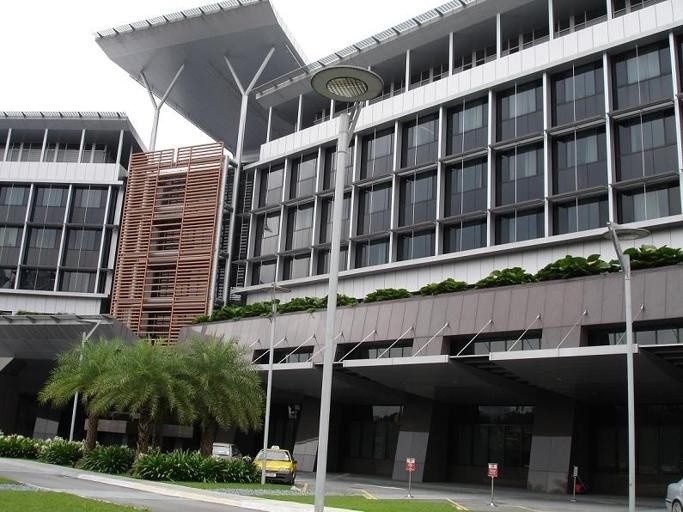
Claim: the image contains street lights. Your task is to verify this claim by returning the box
[309,64,383,511]
[599,221,651,510]
[260,282,291,485]
[67,313,116,443]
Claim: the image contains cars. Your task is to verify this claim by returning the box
[664,477,683,511]
[197,443,242,461]
[251,445,296,484]
